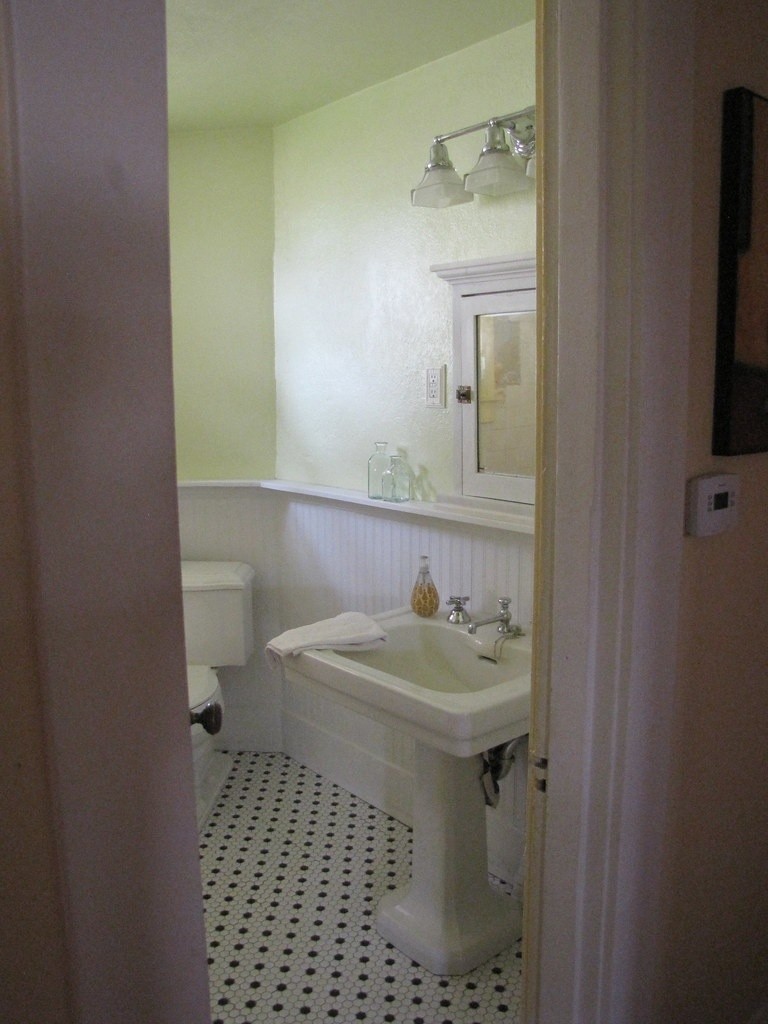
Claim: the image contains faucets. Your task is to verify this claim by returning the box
[467,597,527,639]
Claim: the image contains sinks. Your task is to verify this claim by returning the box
[285,604,529,758]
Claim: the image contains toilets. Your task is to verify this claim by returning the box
[180,560,256,834]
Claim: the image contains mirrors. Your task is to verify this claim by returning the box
[462,289,536,505]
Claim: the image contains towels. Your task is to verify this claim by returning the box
[263,611,390,670]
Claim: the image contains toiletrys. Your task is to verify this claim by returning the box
[411,554,440,619]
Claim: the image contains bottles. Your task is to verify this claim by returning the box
[411,556,439,618]
[381,456,409,503]
[368,442,390,499]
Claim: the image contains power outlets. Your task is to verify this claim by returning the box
[424,364,444,408]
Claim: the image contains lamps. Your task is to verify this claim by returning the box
[410,103,536,207]
